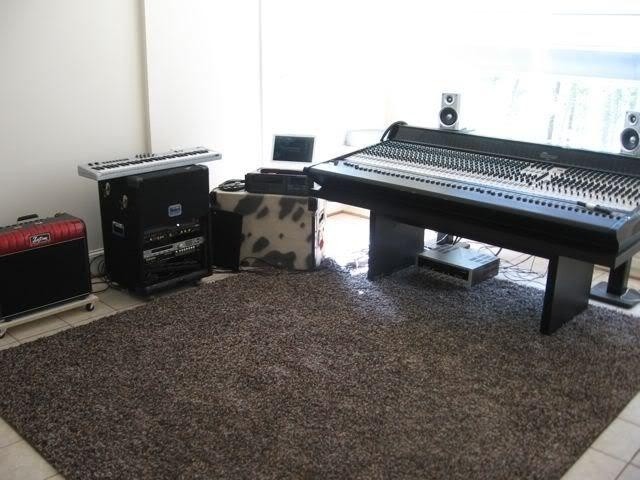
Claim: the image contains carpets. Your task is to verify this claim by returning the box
[0,240,640,479]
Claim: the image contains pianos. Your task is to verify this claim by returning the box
[78,146,222,181]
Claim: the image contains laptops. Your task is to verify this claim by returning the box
[259,134,316,172]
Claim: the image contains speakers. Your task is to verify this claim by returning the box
[618,110,640,156]
[438,92,461,131]
